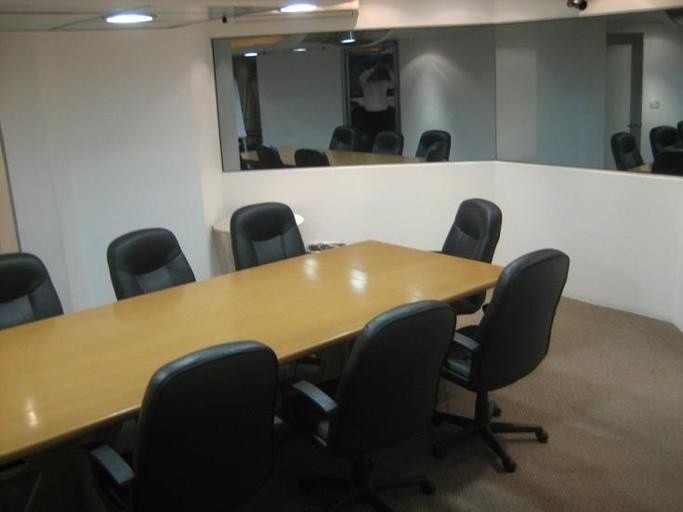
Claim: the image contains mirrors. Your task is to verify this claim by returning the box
[496,7,683,179]
[210,24,496,174]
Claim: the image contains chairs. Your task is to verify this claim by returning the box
[73,342,280,511]
[256,143,283,168]
[433,247,570,471]
[415,129,451,161]
[0,254,63,333]
[231,202,324,388]
[237,137,247,169]
[245,136,264,169]
[424,140,448,162]
[610,119,683,177]
[295,148,330,167]
[279,299,458,512]
[330,126,359,150]
[106,227,195,299]
[372,130,404,155]
[435,197,502,316]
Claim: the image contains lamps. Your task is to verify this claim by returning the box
[340,32,357,44]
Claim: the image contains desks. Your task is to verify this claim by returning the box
[241,145,448,166]
[0,239,503,512]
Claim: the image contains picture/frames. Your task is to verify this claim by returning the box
[339,41,401,153]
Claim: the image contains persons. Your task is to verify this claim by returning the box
[362,62,397,129]
